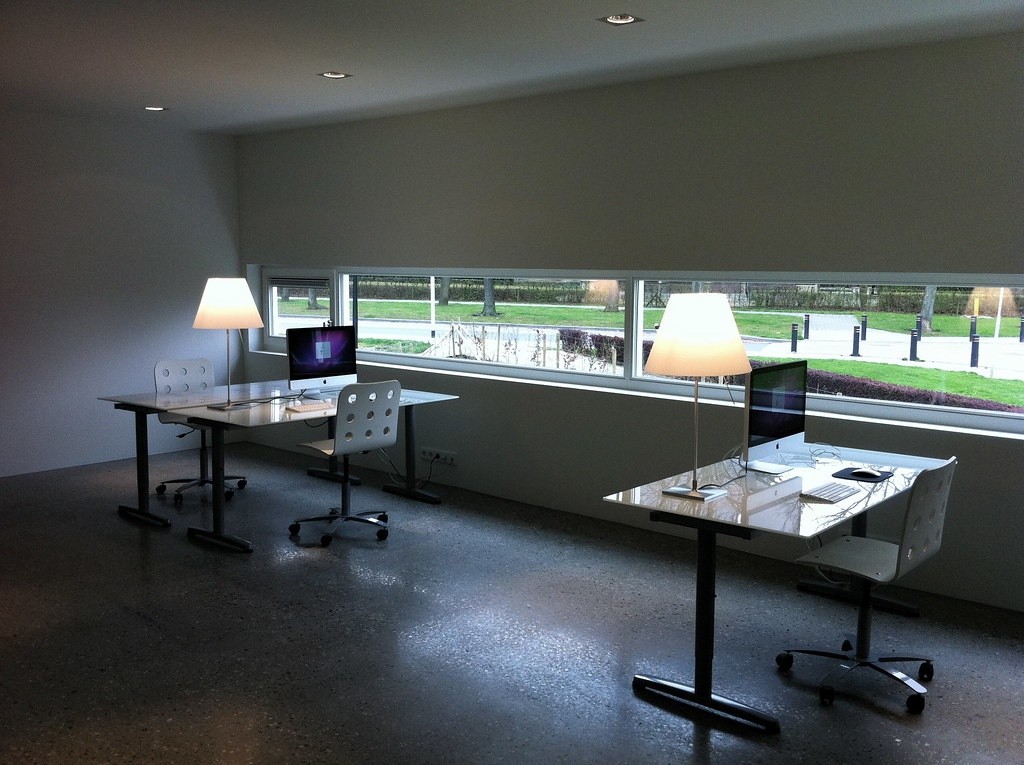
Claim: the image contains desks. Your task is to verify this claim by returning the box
[166,382,459,552]
[602,443,959,737]
[96,380,362,531]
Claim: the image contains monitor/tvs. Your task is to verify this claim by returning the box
[287,326,357,400]
[739,360,808,473]
[743,476,801,535]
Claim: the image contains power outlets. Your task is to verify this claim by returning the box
[420,446,458,466]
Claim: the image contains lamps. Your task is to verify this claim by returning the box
[645,294,753,501]
[192,278,265,412]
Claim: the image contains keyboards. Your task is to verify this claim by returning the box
[799,481,869,506]
[286,403,336,413]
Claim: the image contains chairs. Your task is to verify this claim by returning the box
[288,379,401,548]
[776,455,957,715]
[153,358,247,503]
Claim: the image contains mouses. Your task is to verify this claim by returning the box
[851,468,881,478]
